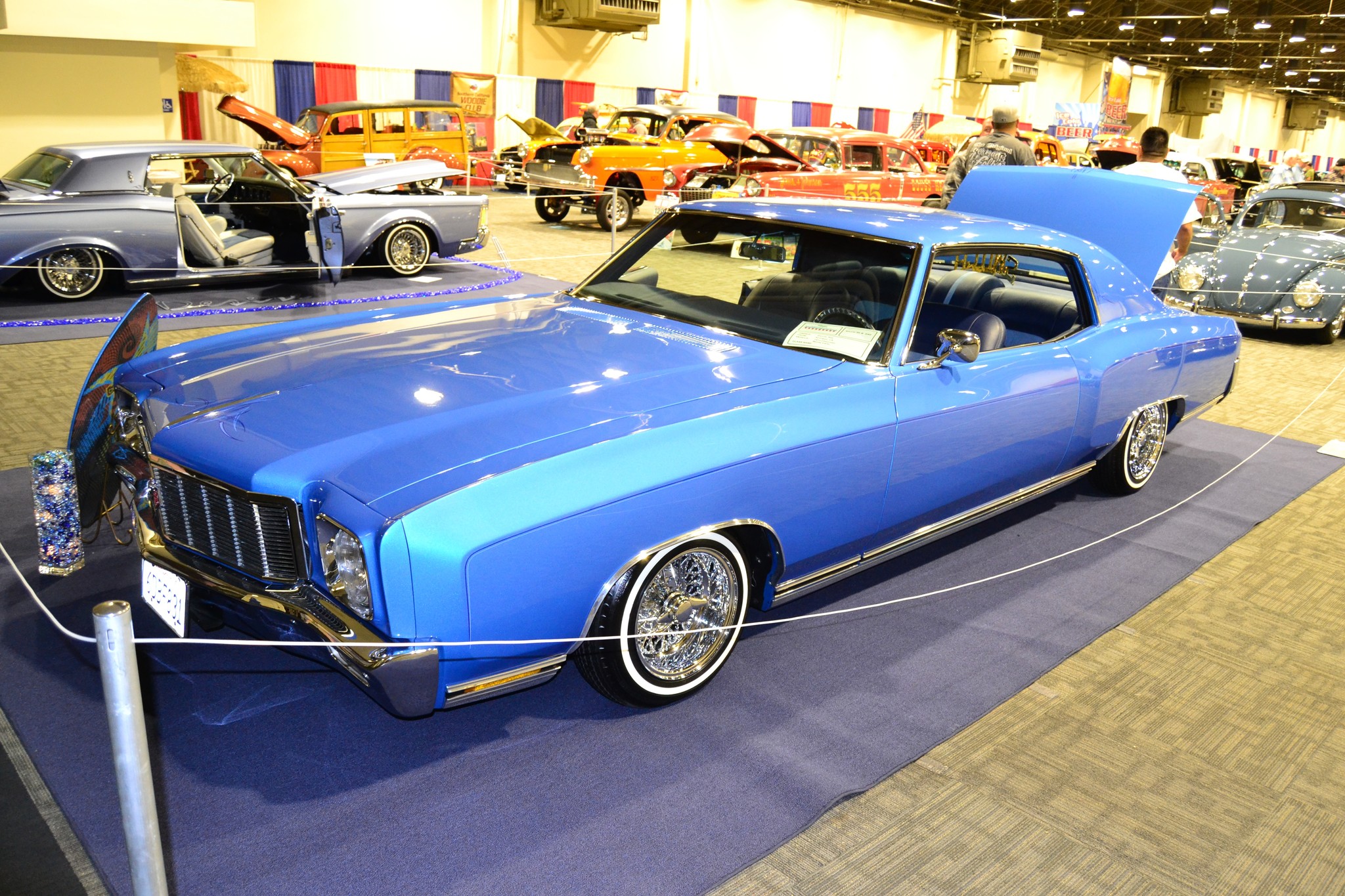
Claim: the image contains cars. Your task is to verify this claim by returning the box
[1015,131,1072,167]
[887,140,955,176]
[0,140,491,303]
[673,128,953,246]
[1170,181,1345,343]
[493,109,686,191]
[61,163,1239,717]
[1064,138,1274,225]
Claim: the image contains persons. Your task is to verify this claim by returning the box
[1268,148,1345,225]
[627,116,648,136]
[943,136,957,153]
[940,116,994,209]
[582,104,600,129]
[965,105,1038,175]
[1113,127,1202,301]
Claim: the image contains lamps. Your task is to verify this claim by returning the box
[1068,0,1336,82]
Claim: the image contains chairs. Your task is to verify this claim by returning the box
[743,260,1080,360]
[145,177,276,265]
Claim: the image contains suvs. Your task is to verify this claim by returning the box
[522,105,756,234]
[216,94,477,191]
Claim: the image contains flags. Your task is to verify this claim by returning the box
[900,108,925,140]
